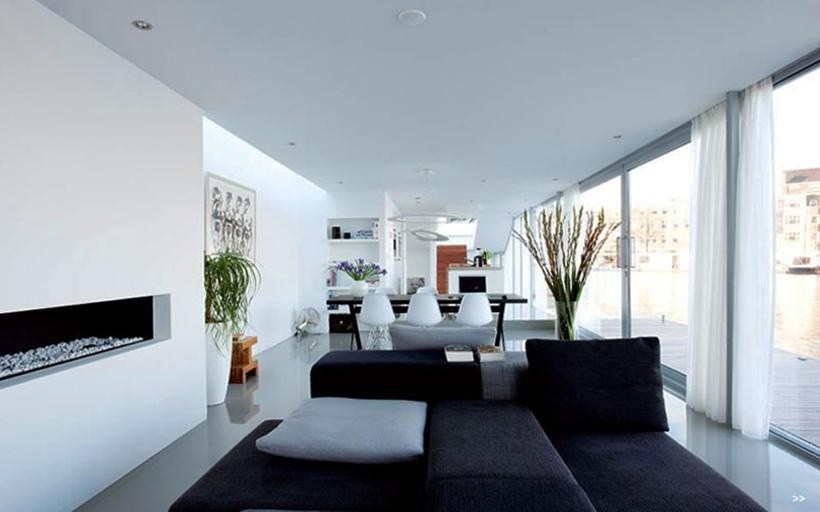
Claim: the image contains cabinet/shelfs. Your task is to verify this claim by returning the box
[327,218,379,291]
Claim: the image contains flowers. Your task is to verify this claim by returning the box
[334,258,386,281]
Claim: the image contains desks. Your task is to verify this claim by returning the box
[326,293,527,350]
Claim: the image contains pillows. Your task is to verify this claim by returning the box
[526,337,669,437]
[256,397,427,462]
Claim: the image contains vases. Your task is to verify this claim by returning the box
[554,300,577,341]
[351,280,368,298]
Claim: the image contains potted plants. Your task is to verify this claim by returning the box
[206,249,258,407]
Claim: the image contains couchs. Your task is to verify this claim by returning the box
[169,348,768,512]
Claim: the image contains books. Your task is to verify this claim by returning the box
[443,341,506,363]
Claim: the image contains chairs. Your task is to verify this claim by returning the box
[361,284,493,350]
